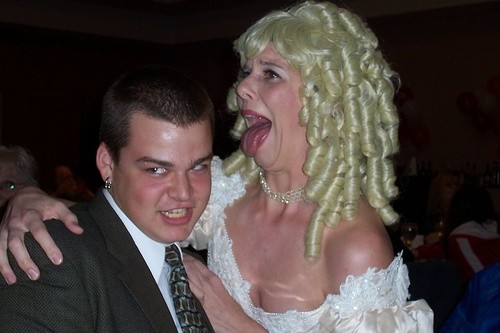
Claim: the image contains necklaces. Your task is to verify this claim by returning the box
[259,167,312,203]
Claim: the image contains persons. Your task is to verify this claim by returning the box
[380,174,500,333]
[0,143,99,225]
[0,65,219,332]
[0,0,435,333]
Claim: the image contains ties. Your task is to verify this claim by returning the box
[164,244,215,331]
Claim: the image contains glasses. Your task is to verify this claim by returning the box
[0,176,19,193]
